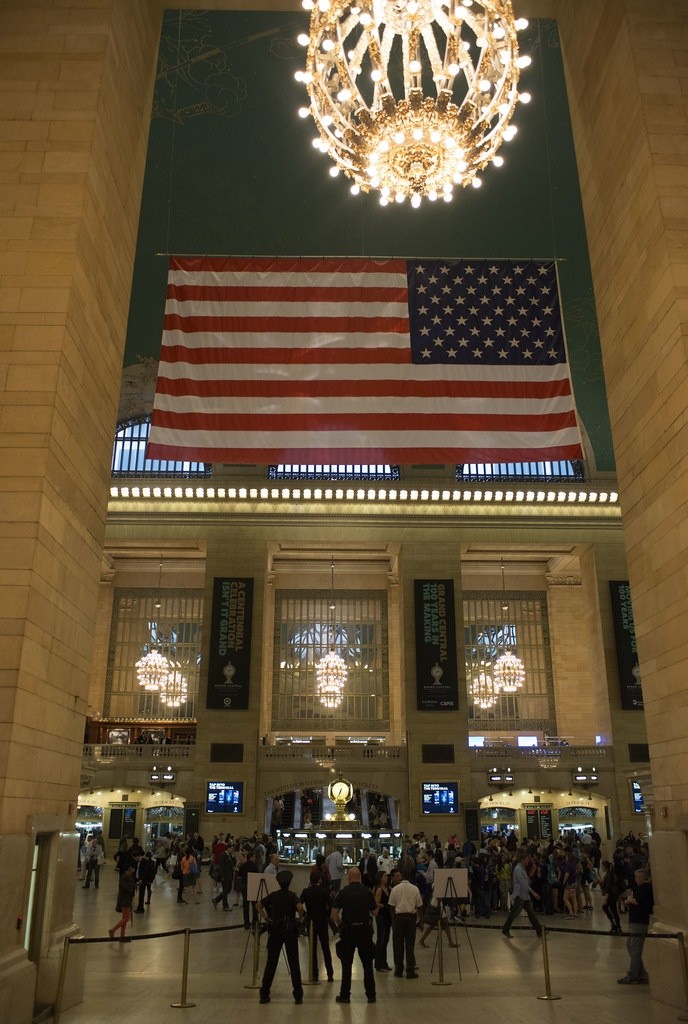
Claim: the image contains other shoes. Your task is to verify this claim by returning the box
[448,944,459,948]
[366,996,377,1004]
[294,996,303,1005]
[617,976,639,985]
[406,974,420,979]
[336,995,350,1003]
[393,971,402,977]
[328,976,333,983]
[418,941,429,948]
[376,966,393,972]
[74,877,258,953]
[537,931,549,937]
[637,976,650,984]
[259,996,271,1005]
[313,977,319,981]
[502,930,513,938]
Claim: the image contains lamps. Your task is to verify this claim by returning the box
[294,0,533,207]
[494,557,525,691]
[467,660,500,709]
[136,599,169,692]
[317,555,347,690]
[317,686,344,707]
[158,672,187,706]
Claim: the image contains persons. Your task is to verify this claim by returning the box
[256,868,306,1005]
[594,832,655,935]
[153,831,205,905]
[615,869,651,985]
[295,871,335,982]
[311,843,401,973]
[388,828,518,981]
[75,826,106,890]
[502,827,602,938]
[331,866,378,1004]
[107,831,159,943]
[209,829,280,930]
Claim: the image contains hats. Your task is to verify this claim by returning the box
[275,870,293,884]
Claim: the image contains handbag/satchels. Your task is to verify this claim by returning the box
[268,918,291,948]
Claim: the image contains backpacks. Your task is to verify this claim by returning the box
[210,861,223,883]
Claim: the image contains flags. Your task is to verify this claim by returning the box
[145,255,589,468]
